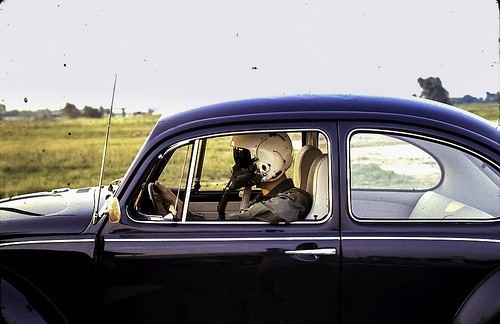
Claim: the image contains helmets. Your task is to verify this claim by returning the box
[230,134,292,183]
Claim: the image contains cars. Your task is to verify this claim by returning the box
[0,70,500,324]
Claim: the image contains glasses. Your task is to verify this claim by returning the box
[233,148,252,168]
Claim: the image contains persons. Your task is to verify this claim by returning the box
[147,132,312,223]
